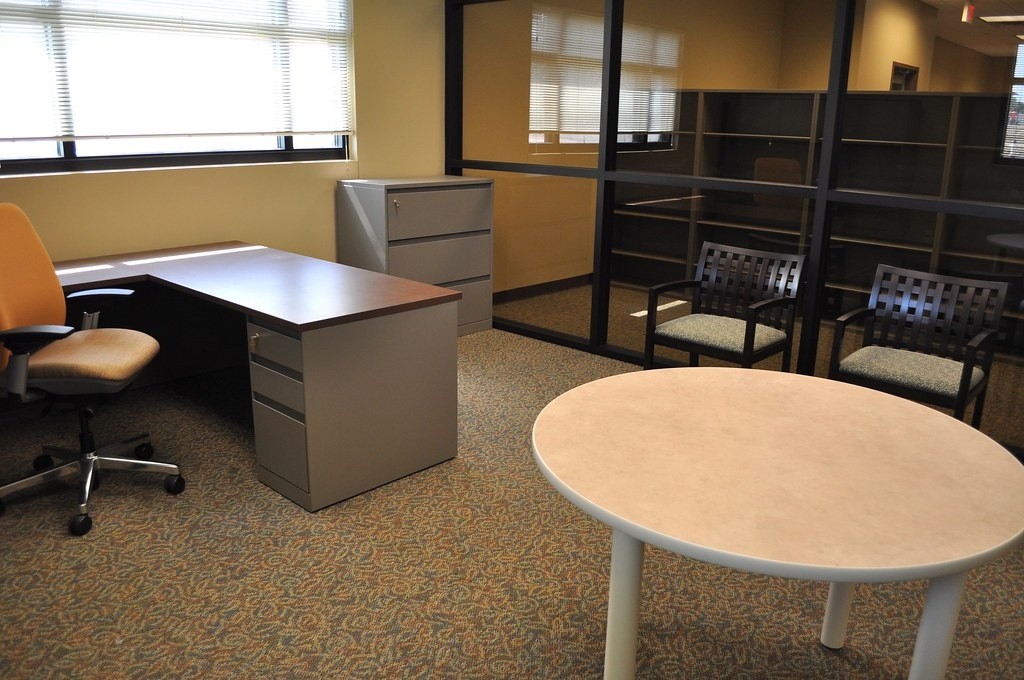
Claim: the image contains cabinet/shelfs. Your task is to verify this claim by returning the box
[335,175,495,335]
[608,87,1024,365]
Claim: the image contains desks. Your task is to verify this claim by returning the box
[52,240,466,514]
[532,363,1024,680]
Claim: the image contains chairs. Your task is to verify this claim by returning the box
[827,263,1011,429]
[0,202,187,537]
[642,241,808,374]
